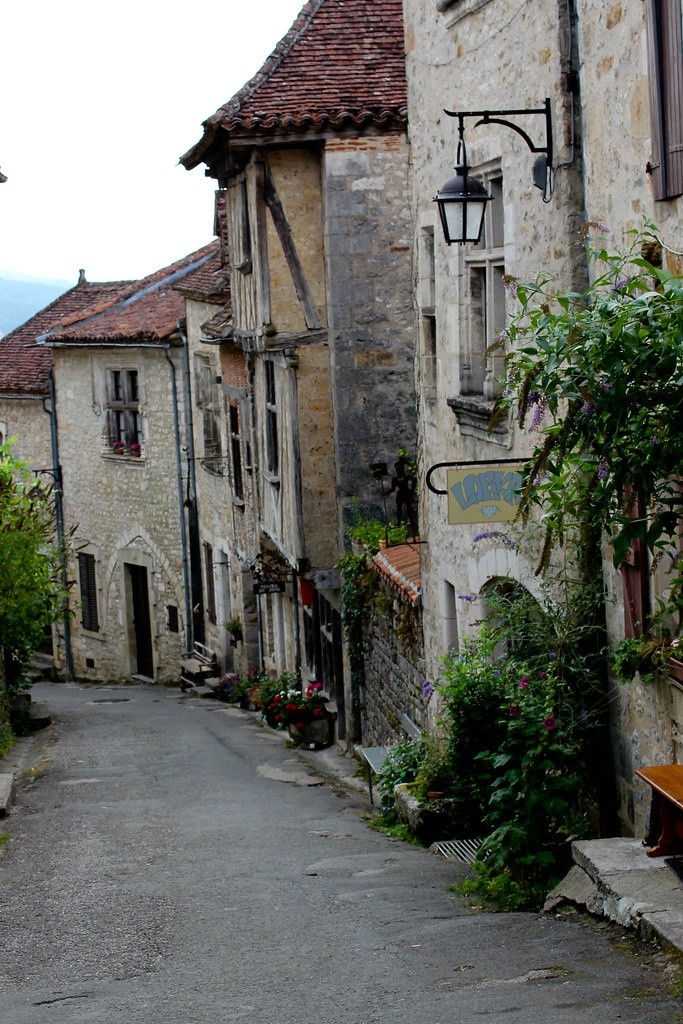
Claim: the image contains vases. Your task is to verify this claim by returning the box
[132,451,140,456]
[116,449,124,455]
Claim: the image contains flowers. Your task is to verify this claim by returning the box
[218,668,337,727]
[130,443,140,451]
[113,441,124,449]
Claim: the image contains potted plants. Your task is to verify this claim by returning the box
[378,526,406,552]
[343,496,382,556]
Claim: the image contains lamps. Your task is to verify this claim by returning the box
[431,98,556,245]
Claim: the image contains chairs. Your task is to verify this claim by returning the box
[362,713,424,806]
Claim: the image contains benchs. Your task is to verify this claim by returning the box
[634,764,683,848]
[176,641,217,692]
